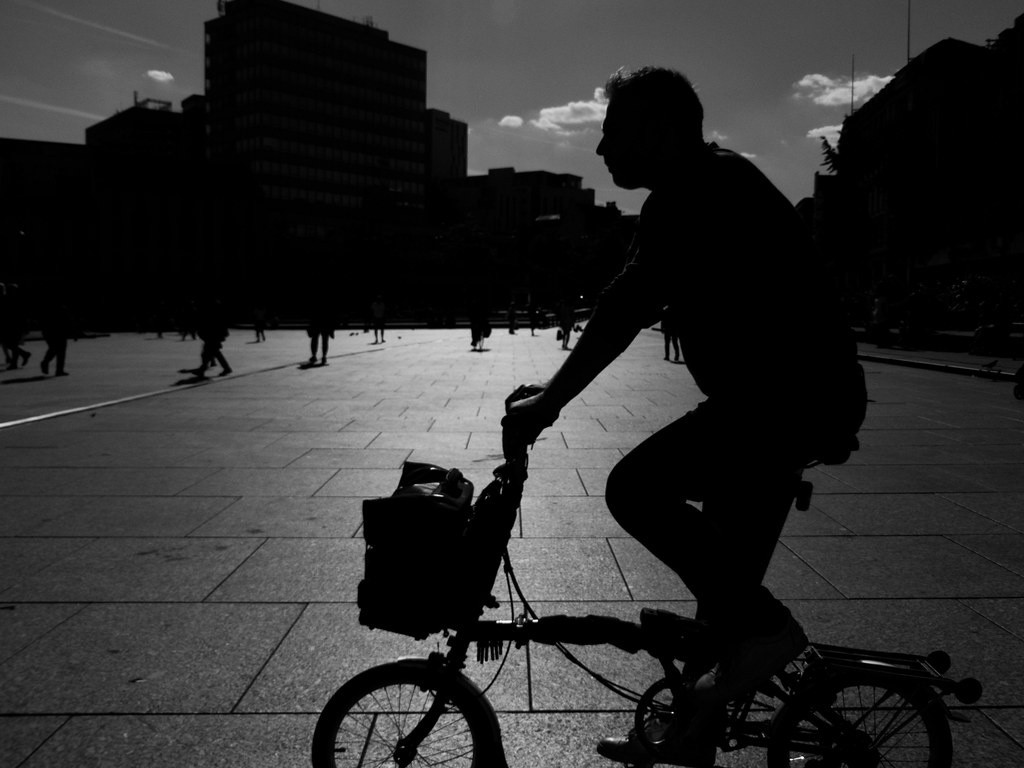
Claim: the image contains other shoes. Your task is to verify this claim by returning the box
[320,356,325,365]
[597,718,715,768]
[310,357,317,364]
[198,365,208,374]
[55,371,70,376]
[683,604,809,705]
[218,368,232,376]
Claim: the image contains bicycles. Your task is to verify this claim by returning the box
[310,383,983,767]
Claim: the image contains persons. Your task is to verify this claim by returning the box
[0,271,1024,380]
[500,67,867,768]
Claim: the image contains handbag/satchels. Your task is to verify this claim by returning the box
[357,459,474,638]
